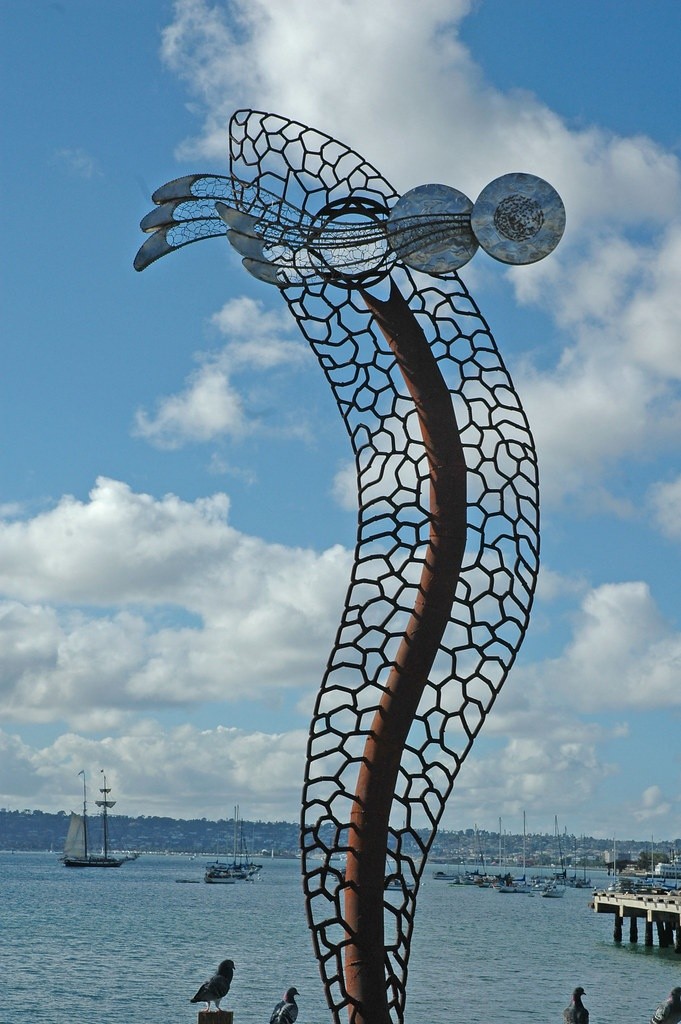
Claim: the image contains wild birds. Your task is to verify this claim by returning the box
[269,988,300,1024]
[564,987,590,1024]
[190,959,235,1014]
[650,987,681,1024]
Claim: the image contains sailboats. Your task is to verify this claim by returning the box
[204,804,264,883]
[59,769,140,867]
[332,853,416,891]
[606,836,678,894]
[433,808,593,898]
